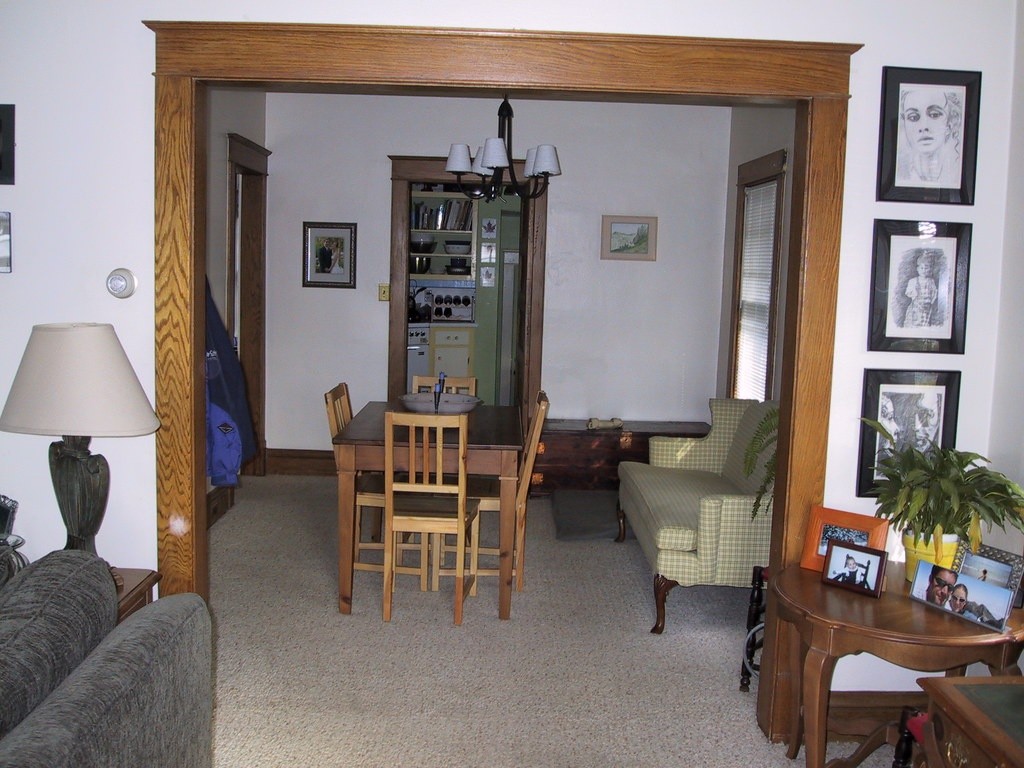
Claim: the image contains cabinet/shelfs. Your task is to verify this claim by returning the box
[530,419,711,500]
[408,191,479,281]
[429,328,474,395]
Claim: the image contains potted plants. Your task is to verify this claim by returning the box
[858,417,1024,583]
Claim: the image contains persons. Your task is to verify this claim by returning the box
[833,557,861,585]
[948,584,977,621]
[319,237,341,274]
[913,565,958,611]
[978,569,987,581]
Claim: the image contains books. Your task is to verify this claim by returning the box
[412,198,472,231]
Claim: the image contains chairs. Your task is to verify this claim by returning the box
[323,376,549,626]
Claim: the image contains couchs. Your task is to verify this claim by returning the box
[0,550,214,768]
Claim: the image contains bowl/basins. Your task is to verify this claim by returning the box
[410,235,471,254]
[445,265,471,275]
[451,259,466,266]
[397,391,481,414]
[409,257,431,274]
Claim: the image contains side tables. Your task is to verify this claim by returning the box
[112,566,162,626]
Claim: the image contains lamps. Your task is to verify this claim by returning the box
[446,94,561,205]
[0,322,161,587]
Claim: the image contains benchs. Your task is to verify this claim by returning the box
[616,398,777,635]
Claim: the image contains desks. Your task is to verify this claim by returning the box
[331,402,523,620]
[772,560,1024,768]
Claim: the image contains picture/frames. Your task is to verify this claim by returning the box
[857,368,961,499]
[301,222,357,289]
[876,66,982,205]
[953,539,1024,620]
[868,218,972,355]
[600,215,657,261]
[799,503,890,598]
